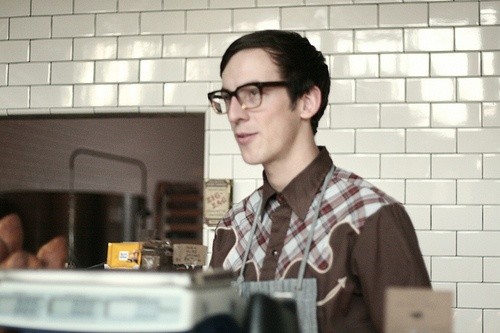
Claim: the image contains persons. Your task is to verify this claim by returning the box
[202,30,436,333]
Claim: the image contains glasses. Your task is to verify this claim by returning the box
[206,81,289,114]
